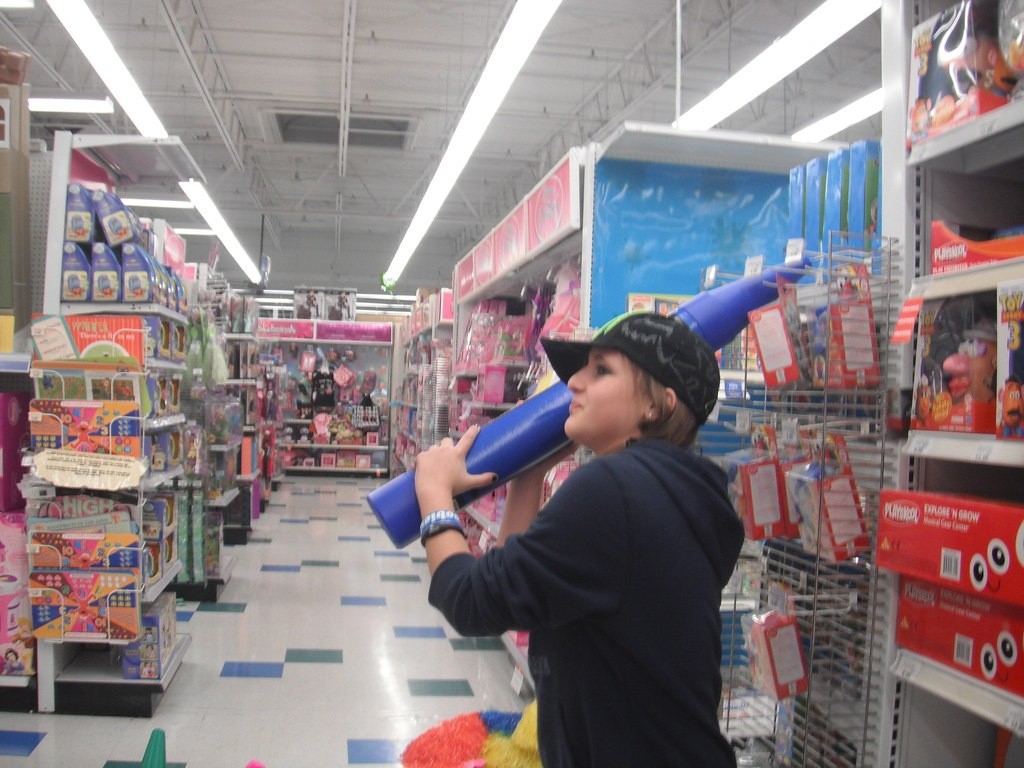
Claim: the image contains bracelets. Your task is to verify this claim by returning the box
[420,510,467,548]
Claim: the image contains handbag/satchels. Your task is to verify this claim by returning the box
[272,344,378,421]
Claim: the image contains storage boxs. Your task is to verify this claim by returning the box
[0,394,38,676]
[62,181,186,680]
[877,489,1024,703]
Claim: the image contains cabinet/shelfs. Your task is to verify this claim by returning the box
[167,131,596,720]
[1,131,192,717]
[876,0,1024,768]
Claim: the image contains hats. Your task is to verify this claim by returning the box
[541,312,720,426]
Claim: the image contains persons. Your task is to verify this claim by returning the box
[414,313,746,768]
